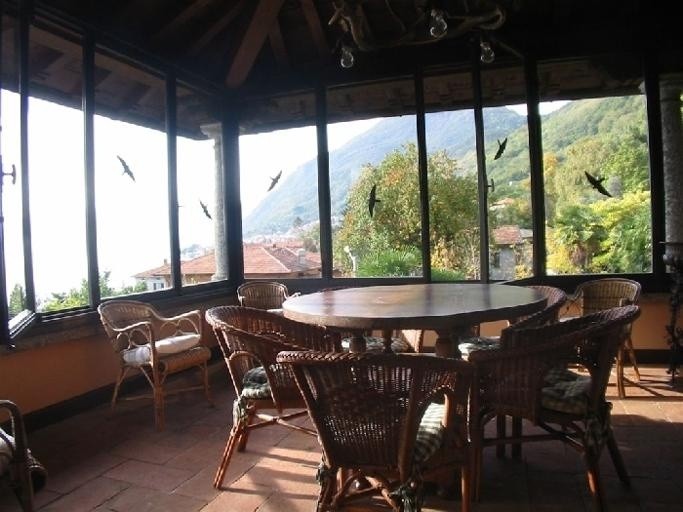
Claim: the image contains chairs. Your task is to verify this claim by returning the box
[0,398,49,511]
[95,299,214,433]
[320,282,429,402]
[234,280,292,338]
[201,302,346,490]
[272,348,501,512]
[453,275,641,510]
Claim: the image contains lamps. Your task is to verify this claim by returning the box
[325,0,527,75]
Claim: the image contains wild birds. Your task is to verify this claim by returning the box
[366,184,381,218]
[584,171,612,198]
[494,137,507,160]
[199,201,212,221]
[116,154,136,182]
[267,170,283,193]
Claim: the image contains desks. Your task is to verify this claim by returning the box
[276,280,551,499]
